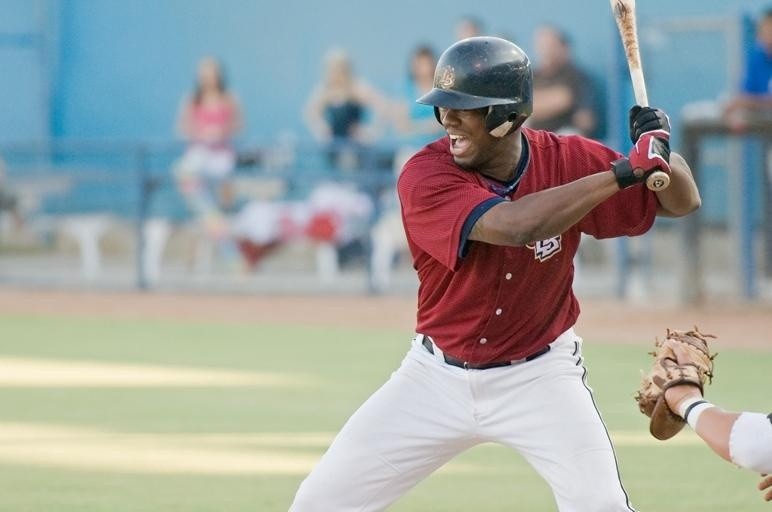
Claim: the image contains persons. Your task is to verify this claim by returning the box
[637,325,771,508]
[301,8,603,290]
[721,8,772,134]
[169,50,240,240]
[287,33,702,512]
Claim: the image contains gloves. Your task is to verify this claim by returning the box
[609,128,673,193]
[626,101,673,144]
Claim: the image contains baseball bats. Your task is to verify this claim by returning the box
[610,0,670,191]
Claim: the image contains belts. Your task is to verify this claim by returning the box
[410,328,552,372]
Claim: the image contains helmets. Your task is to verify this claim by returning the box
[414,34,534,139]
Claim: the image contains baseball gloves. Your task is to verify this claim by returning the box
[635,327,718,440]
